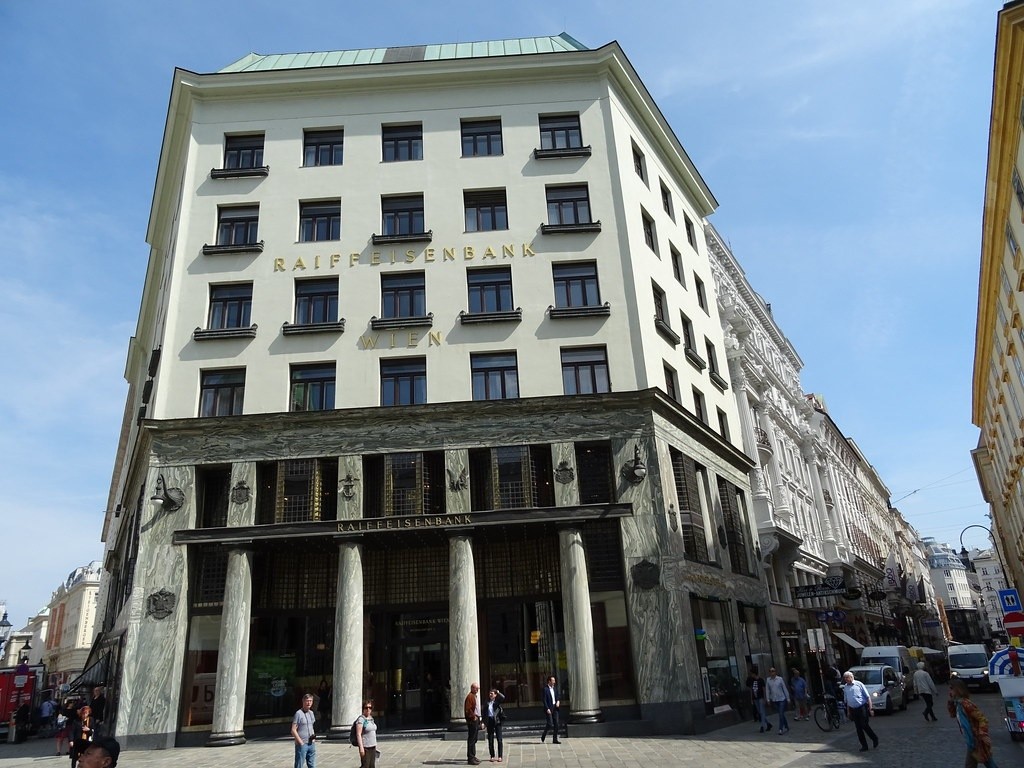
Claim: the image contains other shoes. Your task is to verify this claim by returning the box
[491,757,494,761]
[778,730,783,735]
[873,737,878,748]
[56,752,61,756]
[783,728,790,732]
[553,740,561,744]
[475,758,481,762]
[760,726,764,733]
[541,736,544,742]
[765,723,773,731]
[859,746,868,752]
[468,760,480,765]
[66,752,70,755]
[498,758,502,762]
[922,712,929,721]
[932,718,938,722]
[805,717,810,721]
[794,717,802,721]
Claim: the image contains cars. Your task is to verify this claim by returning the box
[846,664,908,715]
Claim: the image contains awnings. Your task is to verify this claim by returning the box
[828,632,866,650]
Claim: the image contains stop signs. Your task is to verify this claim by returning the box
[1003,611,1024,638]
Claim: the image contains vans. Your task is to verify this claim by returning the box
[947,643,991,692]
[860,646,919,701]
[909,648,927,674]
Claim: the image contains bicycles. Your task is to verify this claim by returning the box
[814,693,842,732]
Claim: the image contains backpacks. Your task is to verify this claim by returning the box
[349,716,365,746]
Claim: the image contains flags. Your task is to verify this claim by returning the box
[883,548,928,603]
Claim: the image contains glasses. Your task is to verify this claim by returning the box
[364,707,373,709]
[769,670,775,672]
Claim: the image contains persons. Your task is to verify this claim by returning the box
[743,661,843,736]
[490,668,571,705]
[464,683,480,765]
[541,675,562,744]
[949,679,998,768]
[14,687,121,768]
[913,662,939,722]
[355,699,377,768]
[481,688,506,763]
[842,671,879,752]
[318,679,332,734]
[290,694,317,768]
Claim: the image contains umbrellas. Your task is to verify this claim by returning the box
[988,647,1024,676]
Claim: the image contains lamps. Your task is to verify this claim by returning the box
[150,473,185,513]
[622,444,647,483]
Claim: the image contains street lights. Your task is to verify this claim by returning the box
[978,586,1012,641]
[15,640,33,710]
[959,525,1010,589]
[871,576,909,645]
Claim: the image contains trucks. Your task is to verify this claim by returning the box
[0,664,54,728]
